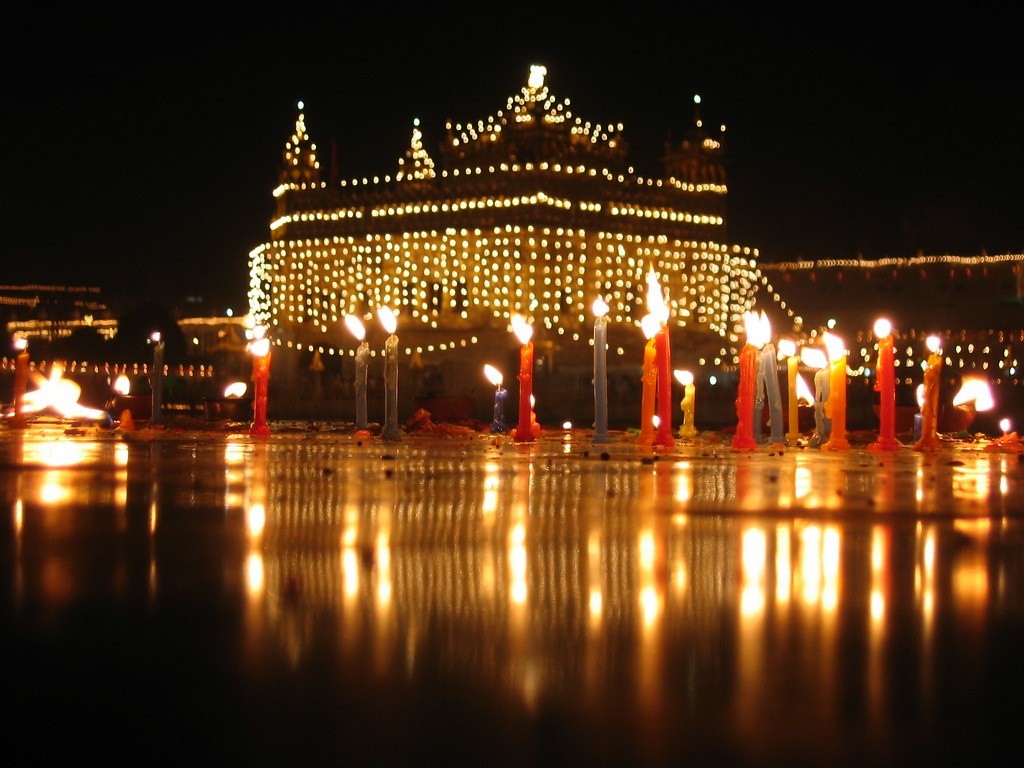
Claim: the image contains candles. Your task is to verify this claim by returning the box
[15,340,30,426]
[483,363,510,434]
[248,337,272,435]
[344,314,369,431]
[913,384,926,444]
[150,332,165,427]
[992,417,1019,452]
[800,346,832,448]
[731,312,758,449]
[865,317,900,452]
[590,300,612,443]
[778,339,803,439]
[529,394,540,439]
[672,368,699,437]
[637,314,662,446]
[646,261,678,447]
[510,313,536,442]
[377,305,402,442]
[913,335,944,451]
[819,332,851,450]
[750,312,765,443]
[760,310,787,446]
